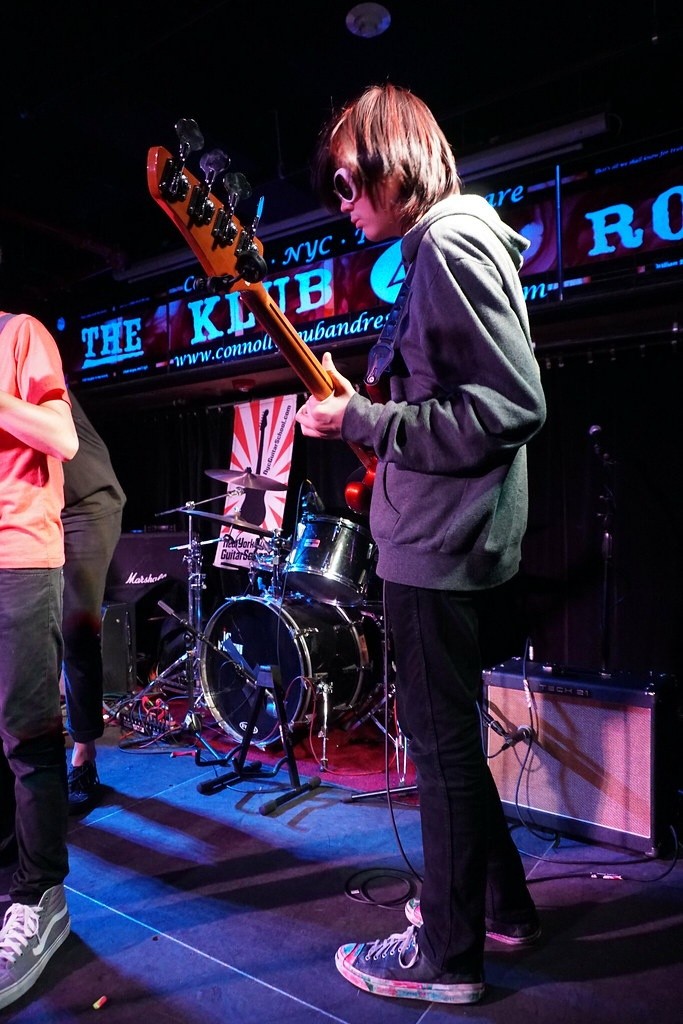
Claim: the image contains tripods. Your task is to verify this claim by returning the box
[337,687,409,796]
[119,488,261,760]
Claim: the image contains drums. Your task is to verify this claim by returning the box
[282,512,379,609]
[195,591,363,752]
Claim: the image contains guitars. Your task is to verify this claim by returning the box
[142,107,390,513]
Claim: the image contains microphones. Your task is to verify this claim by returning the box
[306,479,325,513]
[590,424,608,459]
[500,725,530,750]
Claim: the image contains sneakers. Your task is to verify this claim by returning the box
[404,897,542,946]
[0,883,71,1009]
[67,760,100,809]
[334,924,486,1003]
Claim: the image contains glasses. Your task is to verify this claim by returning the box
[333,166,364,203]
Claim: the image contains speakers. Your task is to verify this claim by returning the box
[101,529,200,697]
[481,656,683,858]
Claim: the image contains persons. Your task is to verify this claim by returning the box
[0,310,79,1008]
[62,389,126,815]
[292,86,547,1004]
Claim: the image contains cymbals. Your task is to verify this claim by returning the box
[180,509,277,540]
[204,465,289,491]
